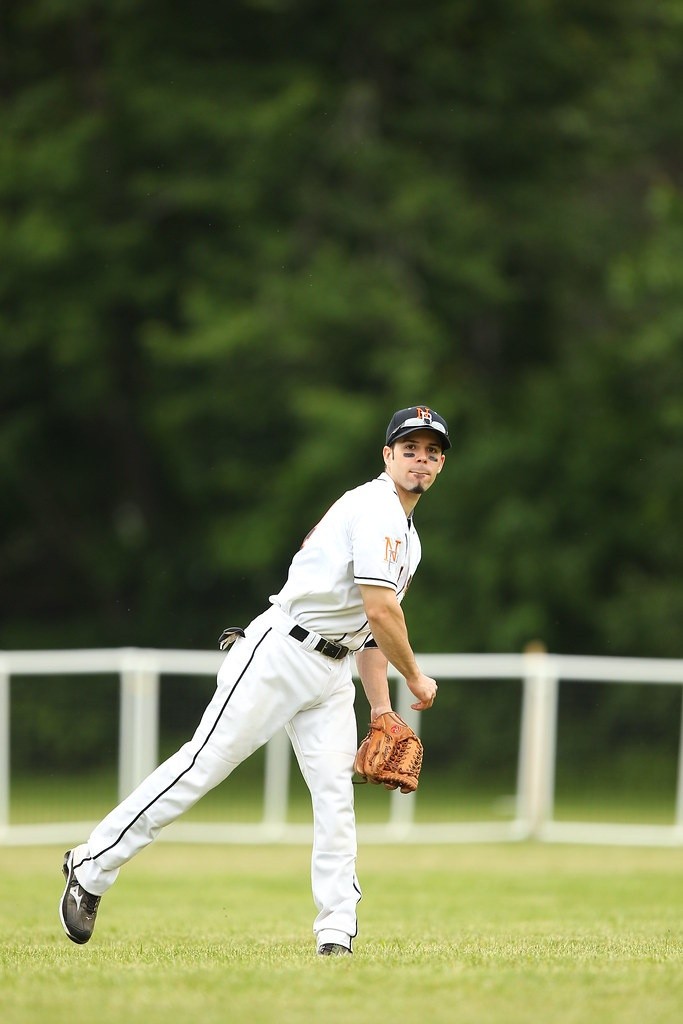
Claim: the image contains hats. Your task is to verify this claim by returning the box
[385,405,452,450]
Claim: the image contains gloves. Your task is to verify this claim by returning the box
[218,626,245,650]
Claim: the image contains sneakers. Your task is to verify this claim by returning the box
[59,849,102,945]
[317,943,353,958]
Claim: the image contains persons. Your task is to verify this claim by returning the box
[59,406,452,957]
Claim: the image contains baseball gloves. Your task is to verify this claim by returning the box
[350,711,424,794]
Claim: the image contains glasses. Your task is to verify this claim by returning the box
[389,417,448,436]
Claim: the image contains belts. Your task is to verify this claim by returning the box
[289,624,349,659]
